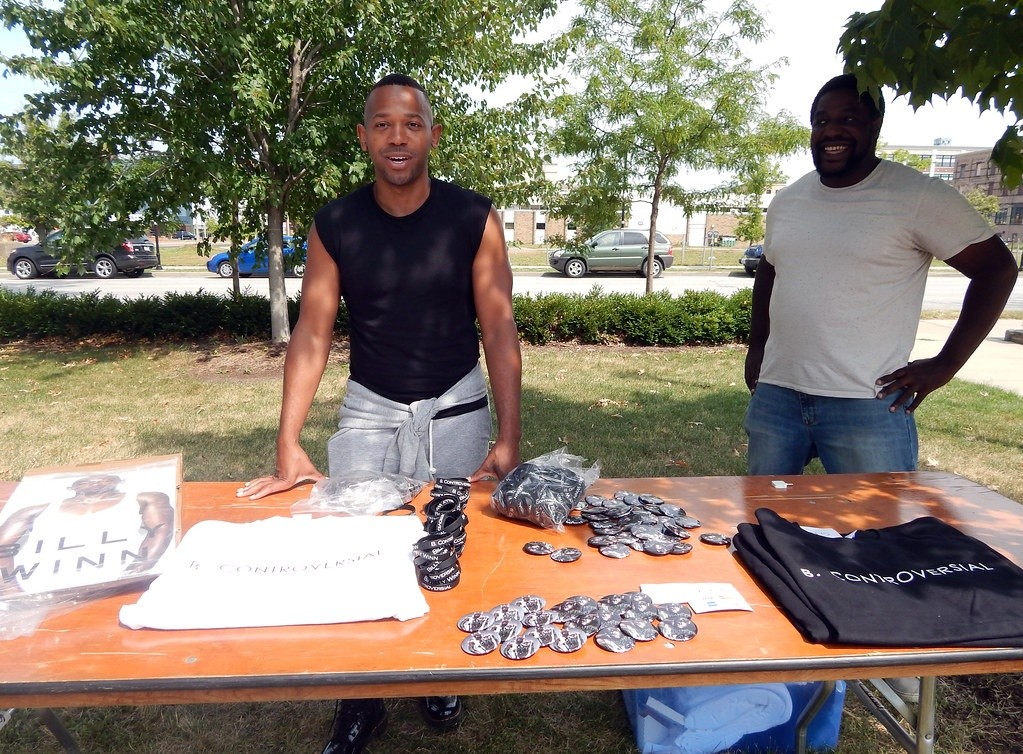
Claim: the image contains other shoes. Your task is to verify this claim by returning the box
[883,677,920,703]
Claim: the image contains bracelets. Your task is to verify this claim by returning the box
[492,463,586,528]
[383,504,416,518]
[414,477,469,591]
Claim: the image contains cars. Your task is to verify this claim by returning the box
[739,244,763,275]
[174,231,196,241]
[207,233,306,278]
[9,232,31,243]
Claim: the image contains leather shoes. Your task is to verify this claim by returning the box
[421,696,461,727]
[322,698,388,754]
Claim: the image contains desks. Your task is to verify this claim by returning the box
[0,471,1023,754]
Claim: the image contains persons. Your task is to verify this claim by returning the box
[236,73,521,754]
[742,73,1018,705]
[457,592,696,660]
[0,473,174,593]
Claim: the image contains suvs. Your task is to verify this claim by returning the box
[549,228,673,279]
[6,227,159,279]
